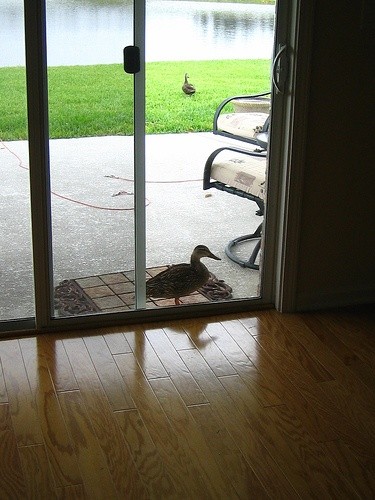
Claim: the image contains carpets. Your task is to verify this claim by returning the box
[54,264,233,317]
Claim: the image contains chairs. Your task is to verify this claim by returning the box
[203,138,268,270]
[213,91,272,149]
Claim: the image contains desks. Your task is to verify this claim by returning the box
[230,98,271,113]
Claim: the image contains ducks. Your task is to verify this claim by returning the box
[132,244,222,305]
[182,73,196,95]
